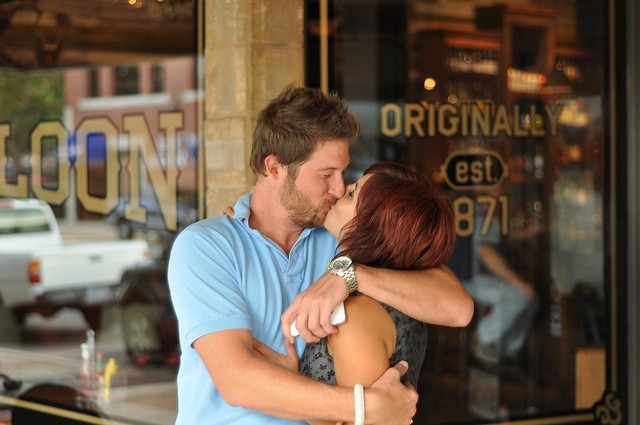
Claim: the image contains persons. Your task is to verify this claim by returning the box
[444,179,539,375]
[219,164,456,425]
[556,126,606,347]
[167,85,474,425]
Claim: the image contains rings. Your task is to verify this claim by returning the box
[410,420,414,424]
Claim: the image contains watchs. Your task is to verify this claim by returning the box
[328,255,358,293]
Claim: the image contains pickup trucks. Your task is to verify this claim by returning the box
[0,197,150,342]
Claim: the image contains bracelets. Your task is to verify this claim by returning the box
[355,384,365,425]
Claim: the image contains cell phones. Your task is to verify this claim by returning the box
[291,302,345,336]
